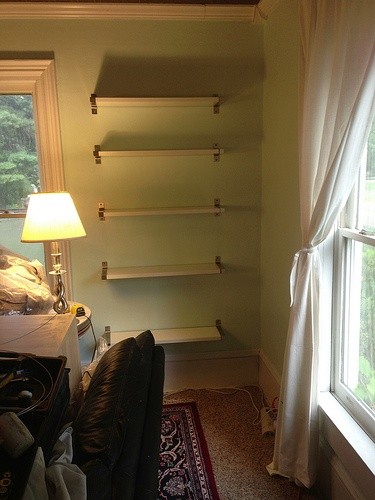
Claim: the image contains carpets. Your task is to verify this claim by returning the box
[158,401,220,500]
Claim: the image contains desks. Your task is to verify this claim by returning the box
[0,314,82,500]
[47,301,92,338]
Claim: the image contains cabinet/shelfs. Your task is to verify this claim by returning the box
[90,94,225,349]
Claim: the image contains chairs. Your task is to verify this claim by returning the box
[73,329,165,500]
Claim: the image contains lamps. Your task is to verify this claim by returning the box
[21,191,88,314]
